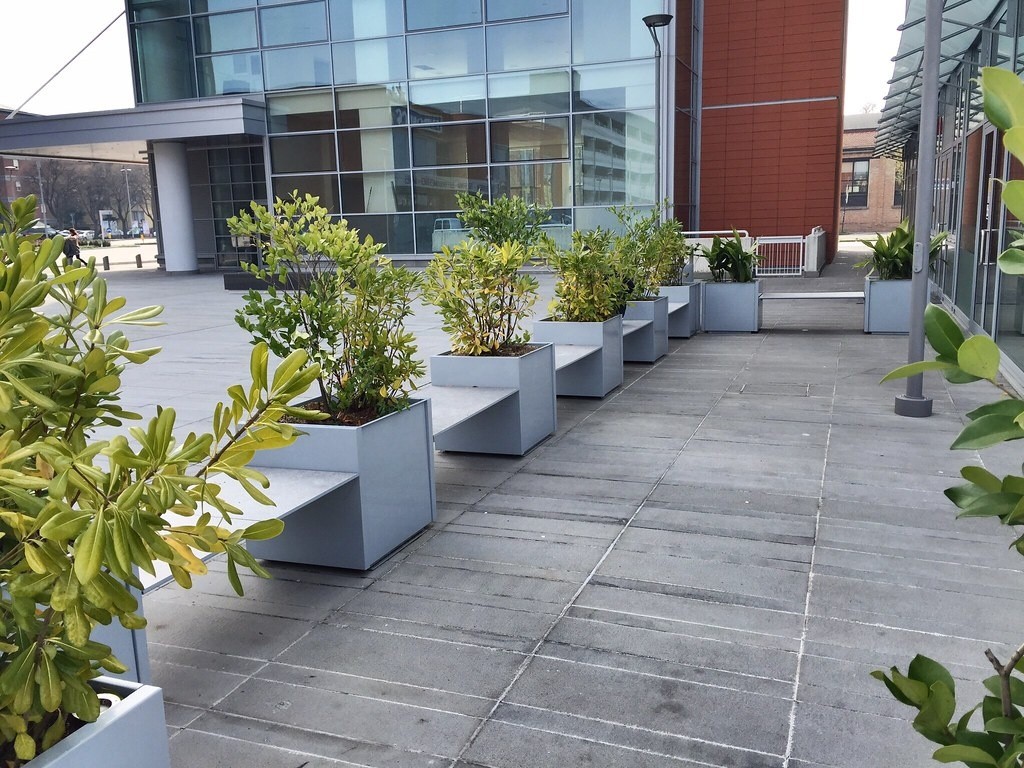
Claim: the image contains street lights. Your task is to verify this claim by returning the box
[120,167,134,239]
[642,14,674,230]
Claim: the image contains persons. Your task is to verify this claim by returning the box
[63,228,88,267]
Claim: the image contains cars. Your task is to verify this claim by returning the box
[60,229,95,242]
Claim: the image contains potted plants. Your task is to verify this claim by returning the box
[531,227,637,398]
[609,202,700,339]
[701,227,768,332]
[612,219,682,362]
[226,187,437,572]
[414,191,551,461]
[852,215,948,335]
